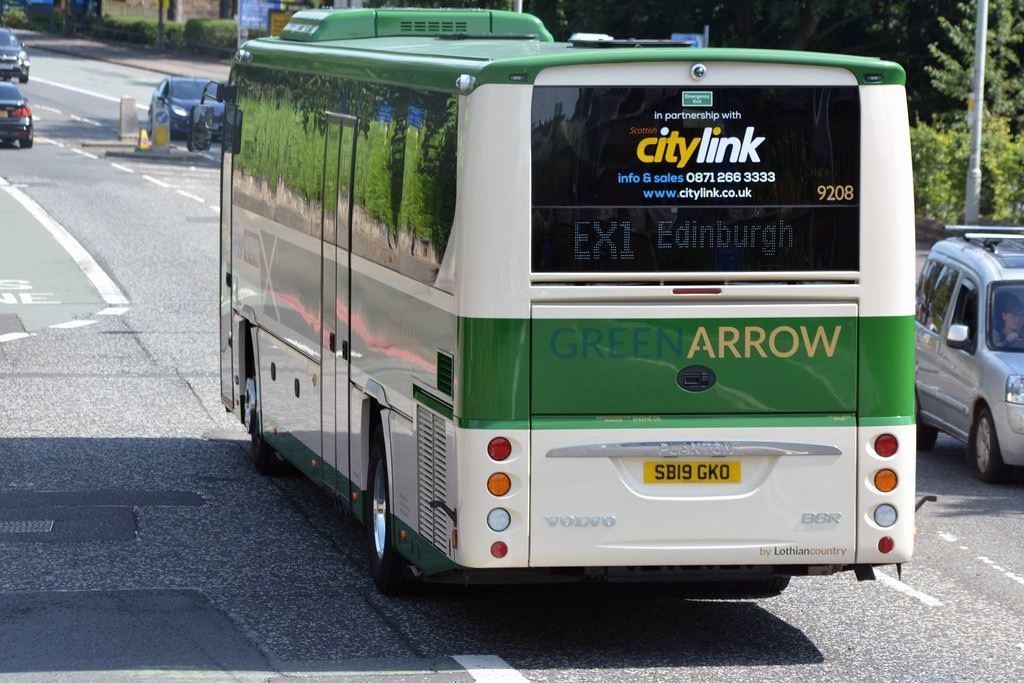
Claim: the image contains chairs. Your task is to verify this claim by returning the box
[992,291,1017,342]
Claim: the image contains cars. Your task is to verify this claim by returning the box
[148,74,228,142]
[23,0,95,28]
[0,80,34,150]
[186,8,917,600]
[0,27,29,83]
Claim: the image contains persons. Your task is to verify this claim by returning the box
[993,299,1024,347]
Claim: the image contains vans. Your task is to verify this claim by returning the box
[917,224,1024,483]
